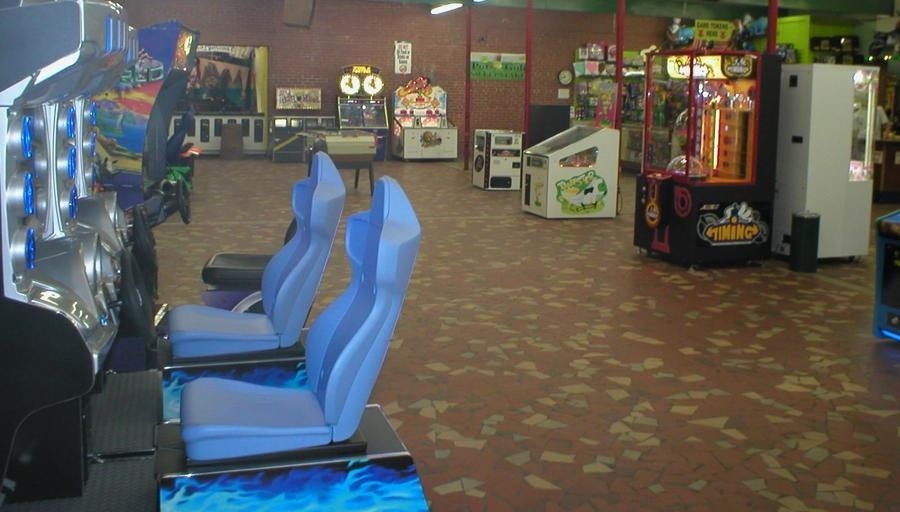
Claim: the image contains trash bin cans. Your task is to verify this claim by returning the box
[790,210,820,272]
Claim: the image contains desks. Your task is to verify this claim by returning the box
[308,154,374,195]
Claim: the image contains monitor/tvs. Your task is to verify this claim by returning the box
[361,104,386,127]
[340,104,363,127]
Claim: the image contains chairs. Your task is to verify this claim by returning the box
[160,175,422,483]
[162,151,346,380]
[203,149,332,308]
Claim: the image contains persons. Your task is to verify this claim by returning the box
[853,88,892,140]
